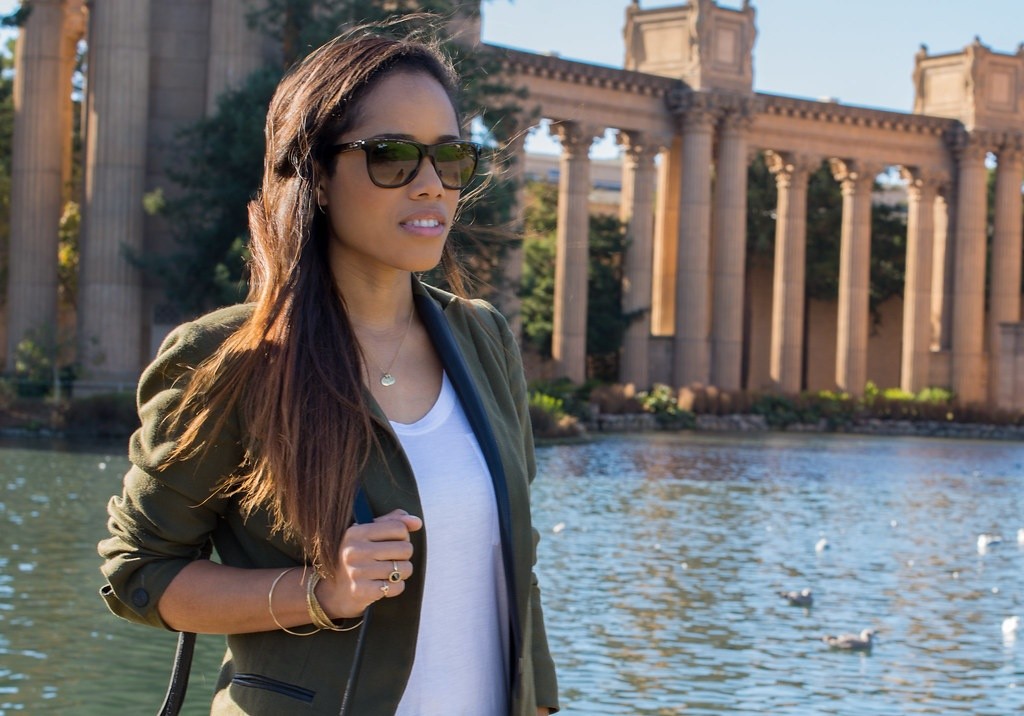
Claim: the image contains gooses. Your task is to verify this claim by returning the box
[776,589,813,605]
[824,630,881,651]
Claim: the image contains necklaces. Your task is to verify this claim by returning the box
[362,303,415,387]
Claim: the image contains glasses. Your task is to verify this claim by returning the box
[331,139,481,190]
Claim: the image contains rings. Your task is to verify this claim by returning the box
[388,561,401,582]
[380,580,389,597]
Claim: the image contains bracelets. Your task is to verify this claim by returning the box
[269,567,320,637]
[305,569,364,631]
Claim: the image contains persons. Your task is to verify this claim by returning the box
[96,14,560,716]
